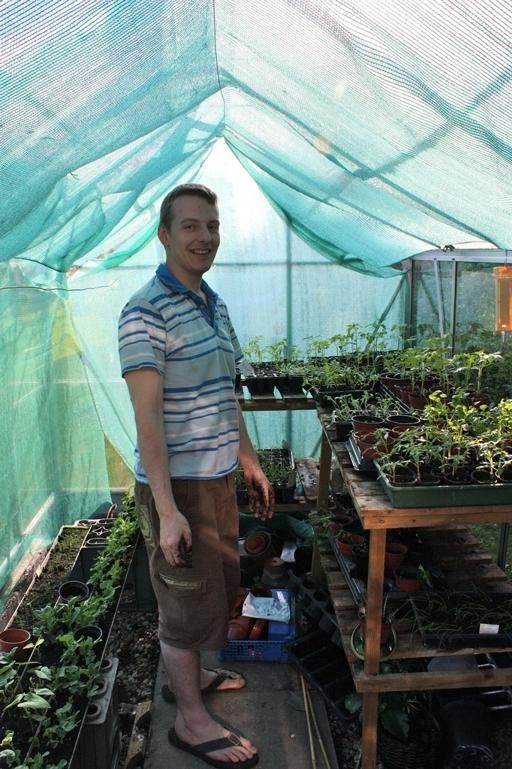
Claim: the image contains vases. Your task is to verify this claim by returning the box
[227,620,249,641]
[74,624,102,664]
[58,581,89,606]
[0,628,31,661]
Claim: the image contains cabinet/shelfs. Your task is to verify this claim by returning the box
[226,386,324,516]
[304,408,512,769]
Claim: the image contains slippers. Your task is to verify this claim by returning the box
[169,722,259,765]
[161,667,246,701]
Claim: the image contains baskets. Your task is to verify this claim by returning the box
[217,584,295,664]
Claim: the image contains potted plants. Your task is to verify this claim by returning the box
[307,492,435,594]
[230,322,511,487]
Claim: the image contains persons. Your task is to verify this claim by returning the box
[119,182,276,768]
